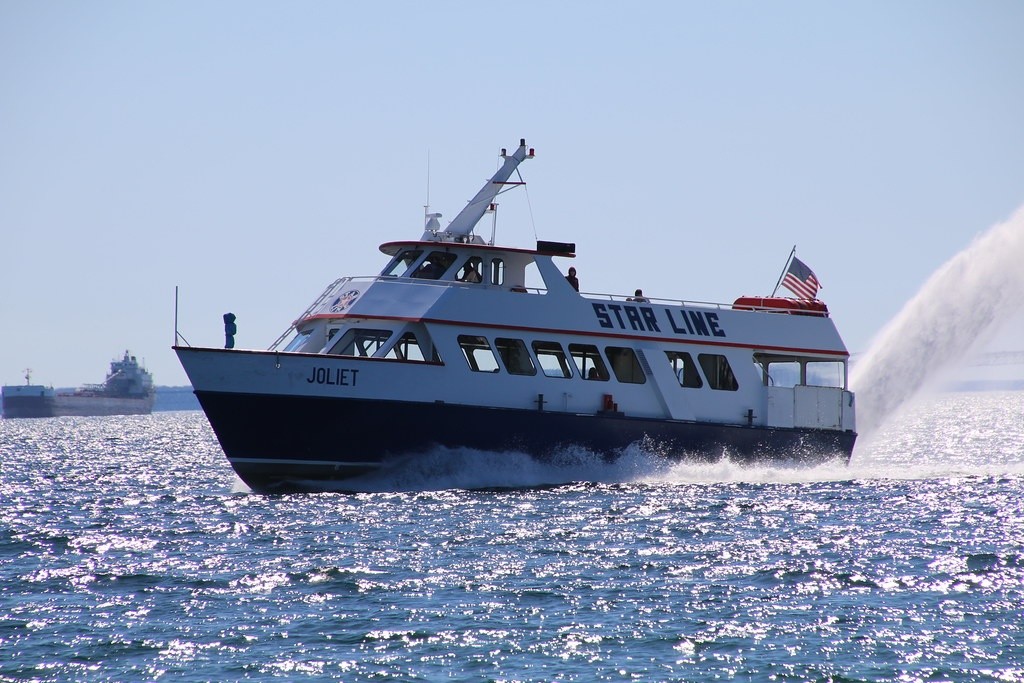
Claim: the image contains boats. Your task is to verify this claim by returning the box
[2,350,158,419]
[171,138,858,496]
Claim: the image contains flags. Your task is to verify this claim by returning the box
[782,257,822,298]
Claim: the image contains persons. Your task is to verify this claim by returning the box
[566,267,578,292]
[697,376,702,386]
[633,289,649,302]
[588,367,598,380]
[421,253,445,275]
[461,262,482,282]
